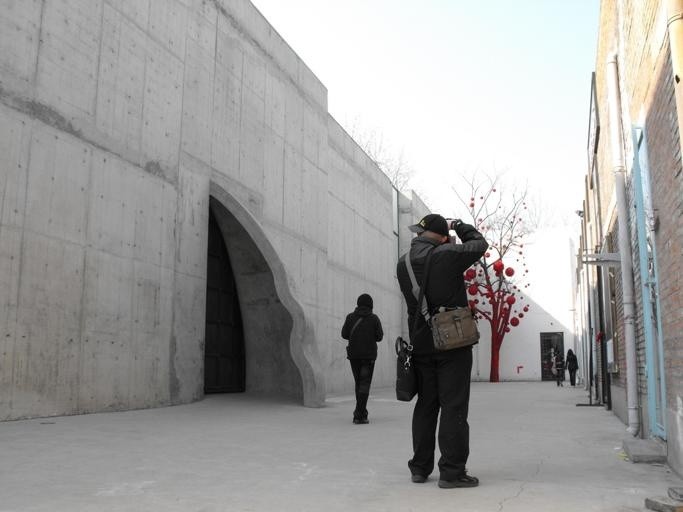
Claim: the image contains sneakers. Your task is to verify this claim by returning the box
[353,410,370,424]
[412,472,428,483]
[438,473,480,488]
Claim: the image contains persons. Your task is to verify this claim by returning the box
[564,349,579,386]
[340,292,384,424]
[394,213,489,489]
[552,349,565,387]
[541,352,558,380]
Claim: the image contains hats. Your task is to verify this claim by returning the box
[408,214,450,236]
[357,294,373,309]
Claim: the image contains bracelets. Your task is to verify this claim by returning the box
[450,218,457,230]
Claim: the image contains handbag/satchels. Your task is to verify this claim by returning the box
[430,307,481,351]
[395,336,417,402]
